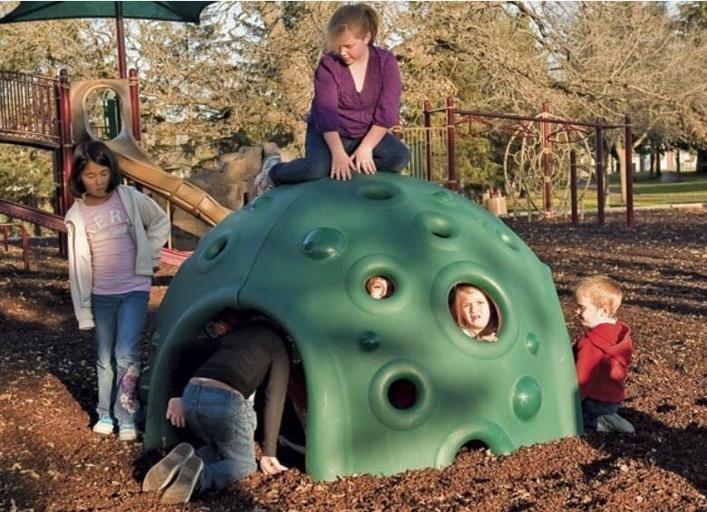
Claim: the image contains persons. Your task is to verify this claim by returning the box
[64,140,172,442]
[142,315,289,506]
[366,274,394,300]
[571,275,635,435]
[165,307,241,428]
[252,3,413,194]
[449,285,499,343]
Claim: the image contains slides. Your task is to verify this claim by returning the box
[0,199,196,268]
[71,77,237,228]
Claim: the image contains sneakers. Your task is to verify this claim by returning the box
[140,440,195,493]
[159,454,204,507]
[251,155,283,196]
[117,421,138,441]
[92,414,117,435]
[596,410,638,434]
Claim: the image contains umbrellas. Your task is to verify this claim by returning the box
[0,1,217,79]
